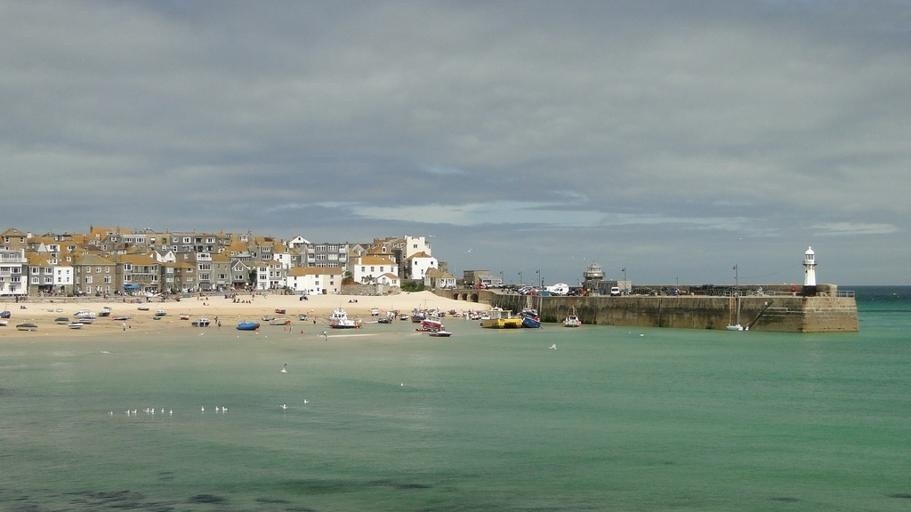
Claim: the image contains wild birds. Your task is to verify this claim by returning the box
[127,408,131,415]
[200,405,205,412]
[169,408,174,415]
[280,403,288,410]
[303,399,310,405]
[134,408,138,414]
[222,405,229,413]
[280,367,288,373]
[151,407,155,414]
[549,342,557,352]
[161,407,165,413]
[215,406,220,412]
[145,407,150,413]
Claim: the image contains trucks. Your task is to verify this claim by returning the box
[610,287,621,296]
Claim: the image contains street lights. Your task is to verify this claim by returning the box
[535,269,542,287]
[621,264,629,289]
[518,271,523,285]
[732,264,738,293]
[500,271,504,283]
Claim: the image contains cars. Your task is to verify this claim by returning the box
[668,287,680,294]
[472,279,581,296]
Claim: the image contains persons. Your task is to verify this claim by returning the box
[215,316,222,327]
[21,304,27,309]
[349,298,358,304]
[121,320,127,336]
[224,293,255,303]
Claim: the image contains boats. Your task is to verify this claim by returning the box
[724,323,748,331]
[518,306,542,328]
[478,303,523,328]
[559,304,582,327]
